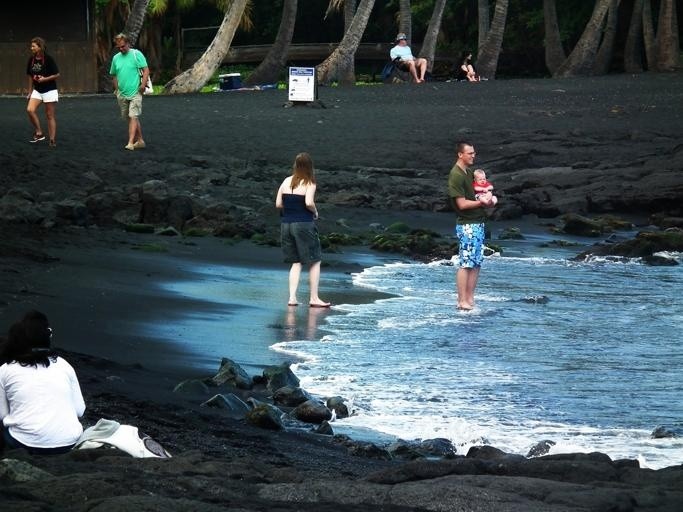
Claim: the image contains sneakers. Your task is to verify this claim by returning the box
[125,144,134,150]
[29,134,46,142]
[49,139,56,147]
[133,141,145,149]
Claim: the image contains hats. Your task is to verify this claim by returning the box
[397,33,406,40]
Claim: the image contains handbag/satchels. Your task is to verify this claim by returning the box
[141,69,153,95]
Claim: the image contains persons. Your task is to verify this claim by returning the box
[0,310,87,456]
[457,49,478,83]
[285,306,325,343]
[390,31,428,84]
[109,33,150,151]
[447,140,498,310]
[25,36,60,148]
[276,152,332,308]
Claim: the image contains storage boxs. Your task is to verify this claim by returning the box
[220,73,242,90]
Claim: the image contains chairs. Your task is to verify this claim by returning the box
[386,45,414,83]
